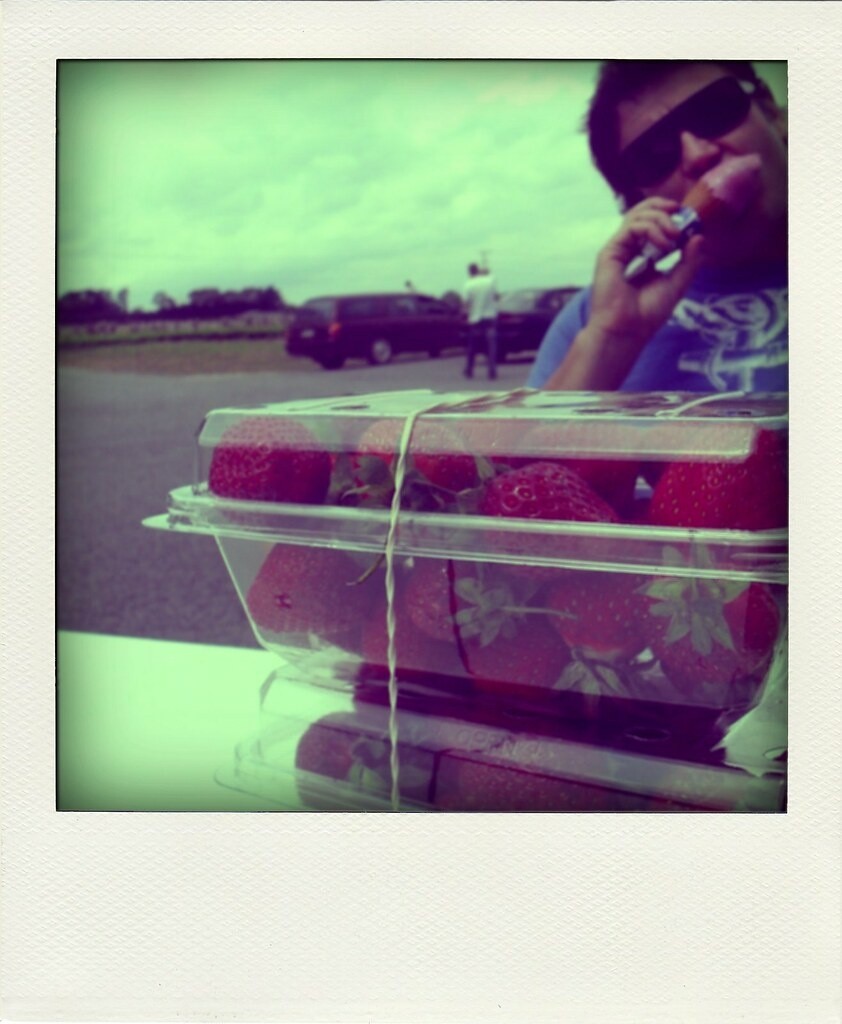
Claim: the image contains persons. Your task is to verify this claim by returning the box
[458,265,503,379]
[524,58,789,400]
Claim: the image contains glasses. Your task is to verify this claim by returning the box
[617,75,759,187]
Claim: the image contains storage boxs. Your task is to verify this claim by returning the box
[144,394,789,811]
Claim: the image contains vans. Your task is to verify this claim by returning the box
[285,291,469,370]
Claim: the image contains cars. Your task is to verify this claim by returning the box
[460,285,583,361]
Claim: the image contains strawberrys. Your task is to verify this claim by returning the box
[204,397,789,813]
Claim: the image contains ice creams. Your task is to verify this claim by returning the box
[678,153,763,231]
